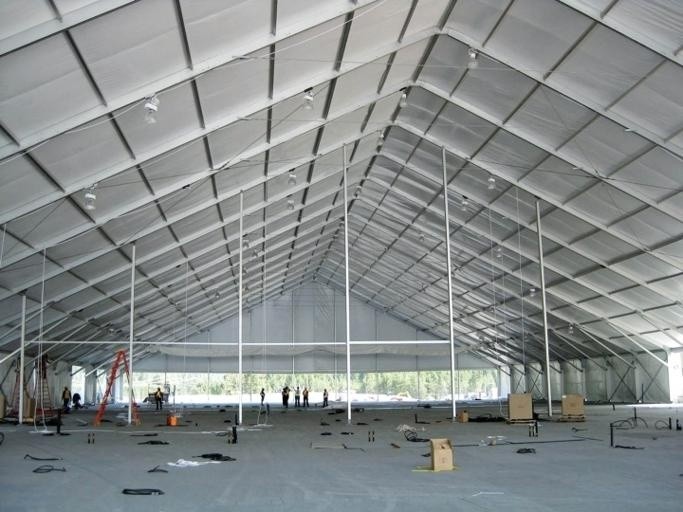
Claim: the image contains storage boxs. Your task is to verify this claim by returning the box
[507,392,533,421]
[560,393,585,416]
[429,438,455,470]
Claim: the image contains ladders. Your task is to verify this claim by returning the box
[95,351,141,427]
[33,370,53,418]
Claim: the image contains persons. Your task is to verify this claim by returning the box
[260,388,265,406]
[323,389,328,407]
[62,386,71,407]
[155,388,163,410]
[282,386,309,408]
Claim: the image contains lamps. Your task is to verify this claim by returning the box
[467,48,478,72]
[287,87,315,211]
[142,96,160,124]
[461,176,575,338]
[399,87,408,109]
[83,181,99,210]
[376,130,385,147]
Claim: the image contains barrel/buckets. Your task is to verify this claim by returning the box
[166,416,178,426]
[459,412,469,424]
